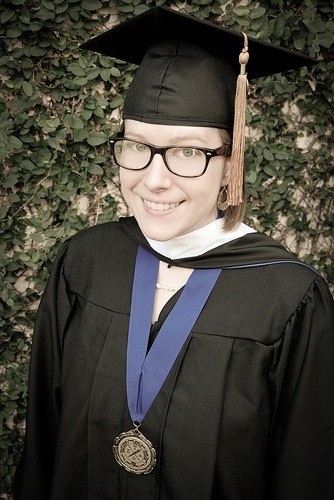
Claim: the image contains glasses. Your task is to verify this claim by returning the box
[106,132,232,177]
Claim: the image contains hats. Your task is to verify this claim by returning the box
[79,5,318,205]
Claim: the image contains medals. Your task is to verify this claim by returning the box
[112,419,156,475]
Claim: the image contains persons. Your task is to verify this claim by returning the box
[7,6,334,500]
